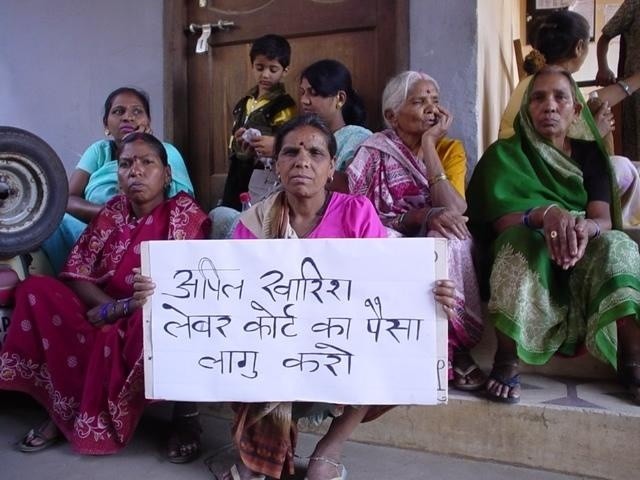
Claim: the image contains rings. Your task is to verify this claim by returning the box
[610,120,614,126]
[550,230,559,240]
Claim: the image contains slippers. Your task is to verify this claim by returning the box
[299,457,348,480]
[219,461,269,480]
[166,407,204,463]
[485,347,525,402]
[450,345,489,392]
[16,418,63,452]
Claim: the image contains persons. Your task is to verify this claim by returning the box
[344,69,488,391]
[36,87,196,275]
[132,113,457,479]
[219,34,298,212]
[0,132,211,464]
[497,12,640,230]
[595,0,640,161]
[299,57,374,172]
[464,64,640,404]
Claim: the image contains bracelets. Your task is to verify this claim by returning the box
[100,296,132,323]
[587,218,600,241]
[617,80,633,96]
[428,172,449,189]
[523,208,542,229]
[543,203,561,221]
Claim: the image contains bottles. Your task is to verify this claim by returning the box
[586,91,614,156]
[239,191,252,213]
